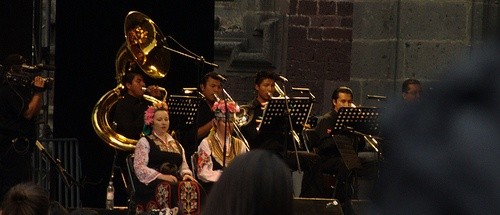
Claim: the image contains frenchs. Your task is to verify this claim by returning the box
[90,8,173,150]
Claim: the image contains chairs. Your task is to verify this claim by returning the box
[119,150,208,199]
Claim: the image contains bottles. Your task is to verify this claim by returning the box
[106,182,114,210]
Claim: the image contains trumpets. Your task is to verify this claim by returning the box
[267,82,318,129]
[212,91,254,126]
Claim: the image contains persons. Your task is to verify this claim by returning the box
[312,86,383,215]
[0,52,45,200]
[110,71,148,203]
[233,71,318,197]
[134,102,207,215]
[402,79,424,102]
[0,150,294,215]
[197,99,247,194]
[189,72,224,170]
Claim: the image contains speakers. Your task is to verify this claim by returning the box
[350,200,377,215]
[293,197,344,215]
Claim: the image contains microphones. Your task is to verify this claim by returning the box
[279,76,288,82]
[35,64,56,71]
